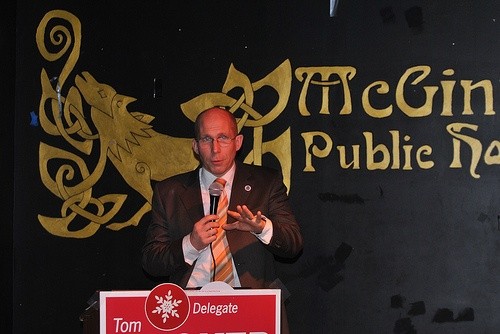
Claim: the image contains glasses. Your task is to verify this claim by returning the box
[198,135,236,145]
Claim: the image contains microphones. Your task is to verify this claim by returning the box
[208,182,224,230]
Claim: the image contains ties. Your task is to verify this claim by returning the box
[211,178,234,289]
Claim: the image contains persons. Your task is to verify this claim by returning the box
[139,105,305,289]
